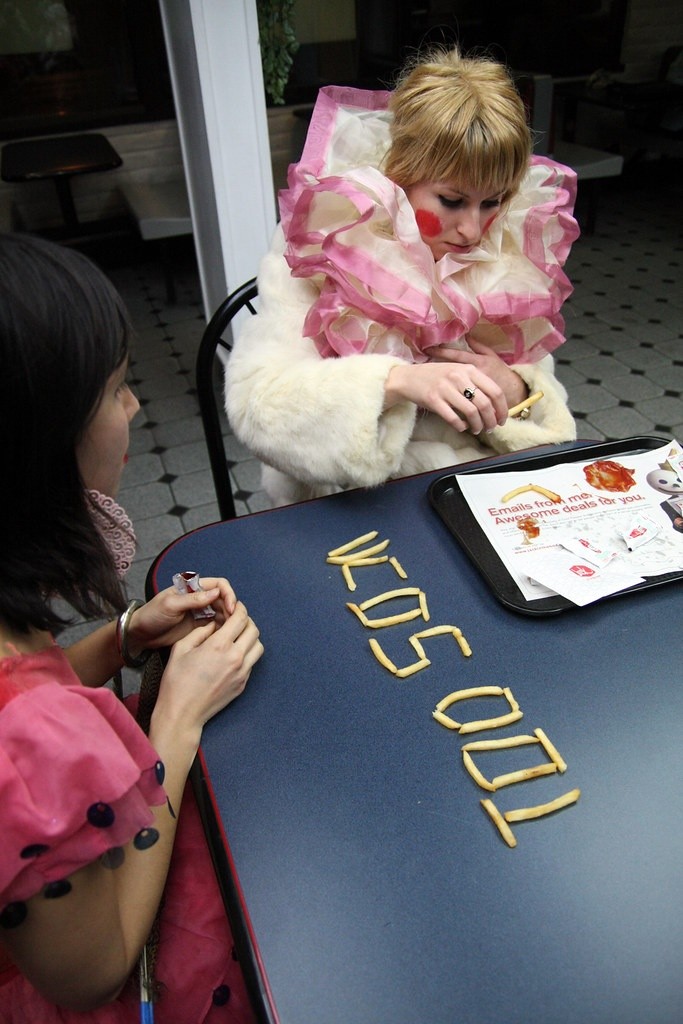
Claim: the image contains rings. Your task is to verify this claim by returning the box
[464,386,477,400]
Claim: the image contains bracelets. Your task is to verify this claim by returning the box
[515,389,532,423]
[116,599,153,670]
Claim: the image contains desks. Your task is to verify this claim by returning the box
[139,438,682,1024]
[555,81,683,173]
[1,133,122,267]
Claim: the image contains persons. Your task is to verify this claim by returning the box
[0,230,263,1023]
[221,46,578,507]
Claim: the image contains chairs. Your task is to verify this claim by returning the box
[194,275,258,520]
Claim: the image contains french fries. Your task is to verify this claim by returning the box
[325,531,581,846]
[502,485,561,503]
[507,390,544,417]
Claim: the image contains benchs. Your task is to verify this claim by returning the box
[513,72,624,221]
[631,45,683,166]
[117,182,192,241]
